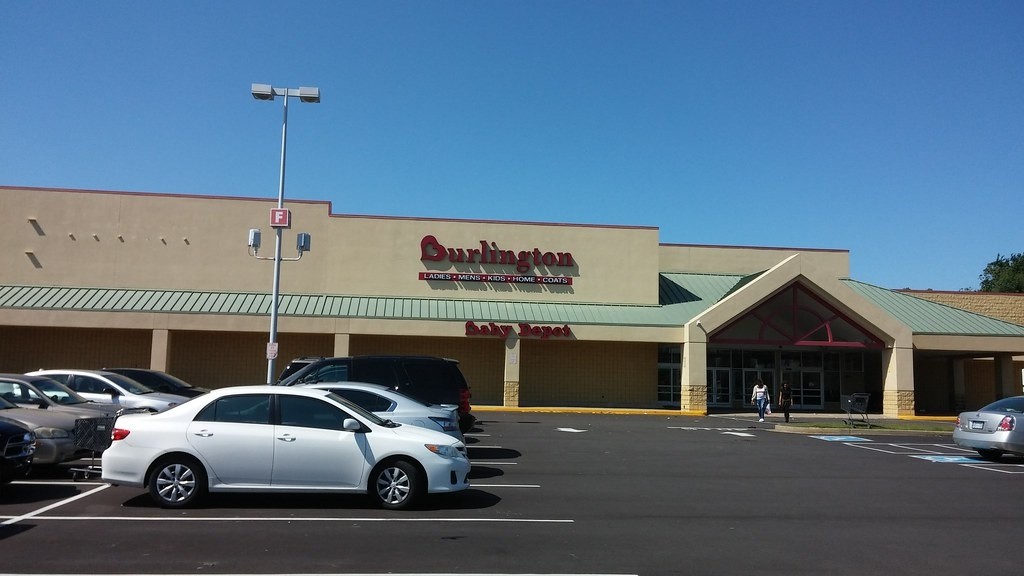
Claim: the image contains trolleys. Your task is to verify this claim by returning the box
[841,393,872,429]
[70,406,150,481]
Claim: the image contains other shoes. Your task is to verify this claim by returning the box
[759,418,764,422]
[785,421,788,423]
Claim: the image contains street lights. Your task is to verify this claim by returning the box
[250,82,321,385]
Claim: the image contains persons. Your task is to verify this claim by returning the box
[778,382,793,423]
[751,379,770,422]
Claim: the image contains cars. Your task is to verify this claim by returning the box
[0,373,124,465]
[100,367,211,399]
[0,415,36,484]
[0,396,79,464]
[13,368,192,413]
[953,396,1024,460]
[298,365,347,384]
[224,381,466,446]
[101,385,471,509]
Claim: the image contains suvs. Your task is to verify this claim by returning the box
[277,357,325,383]
[276,355,471,435]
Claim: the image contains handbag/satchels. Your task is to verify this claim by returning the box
[765,404,771,415]
